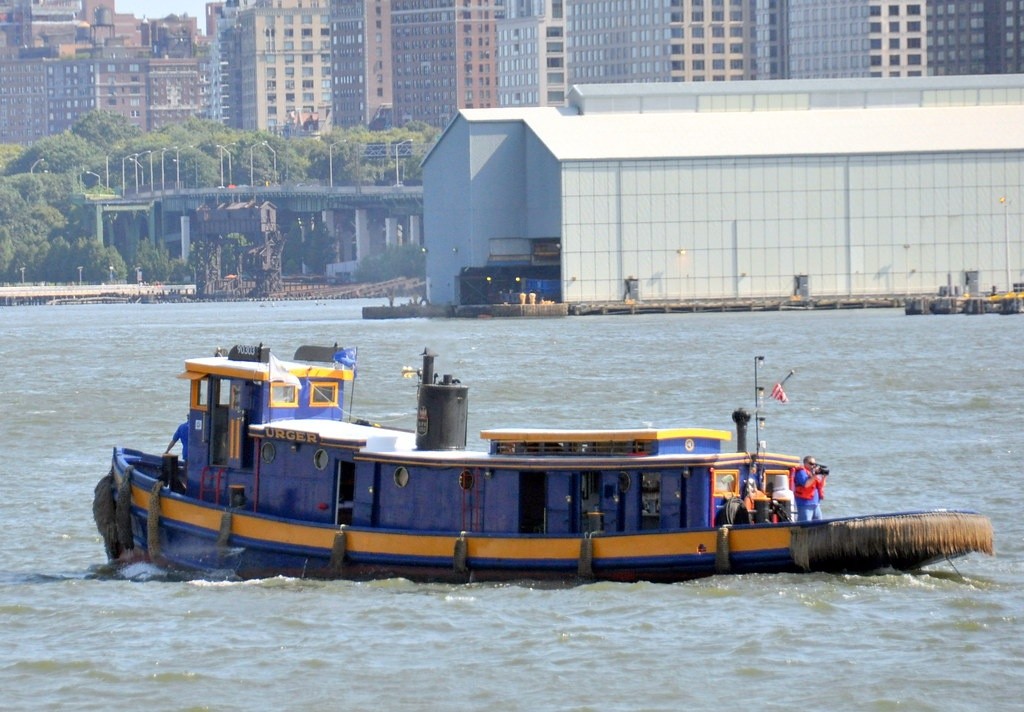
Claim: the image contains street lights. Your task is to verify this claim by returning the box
[195,143,212,189]
[329,139,347,188]
[395,138,414,187]
[177,145,193,190]
[220,142,236,189]
[86,170,101,193]
[135,150,151,196]
[129,157,144,186]
[261,141,276,186]
[161,146,178,191]
[250,141,265,187]
[150,148,166,192]
[217,144,232,185]
[122,153,139,198]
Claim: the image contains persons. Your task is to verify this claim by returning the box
[794,456,827,520]
[164,412,190,486]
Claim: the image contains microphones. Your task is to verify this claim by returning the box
[821,466,827,468]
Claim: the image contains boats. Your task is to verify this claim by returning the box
[88,341,996,595]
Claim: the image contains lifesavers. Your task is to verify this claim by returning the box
[743,492,767,510]
